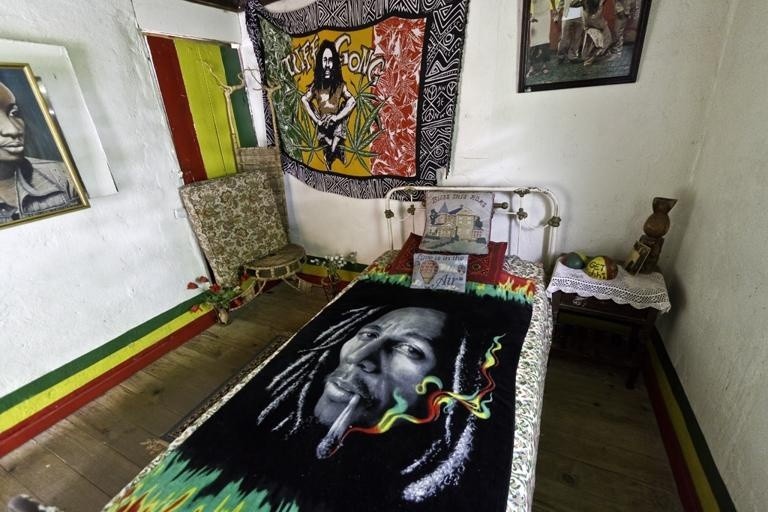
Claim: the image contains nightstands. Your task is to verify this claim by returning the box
[544,250,670,390]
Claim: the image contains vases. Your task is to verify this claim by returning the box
[320,275,338,301]
[208,303,236,325]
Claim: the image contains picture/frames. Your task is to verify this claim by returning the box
[515,0,652,95]
[0,59,92,231]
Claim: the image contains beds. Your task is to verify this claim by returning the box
[9,181,558,511]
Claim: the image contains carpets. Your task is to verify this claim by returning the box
[140,329,291,460]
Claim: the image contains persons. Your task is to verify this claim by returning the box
[0,82,78,222]
[301,39,358,172]
[626,247,642,274]
[526,0,635,81]
[255,301,494,509]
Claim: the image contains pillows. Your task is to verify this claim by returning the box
[386,232,508,286]
[417,188,491,251]
[409,251,469,296]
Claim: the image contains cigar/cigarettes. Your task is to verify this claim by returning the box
[314,394,361,459]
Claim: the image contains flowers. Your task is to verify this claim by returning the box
[308,250,358,275]
[173,270,253,313]
